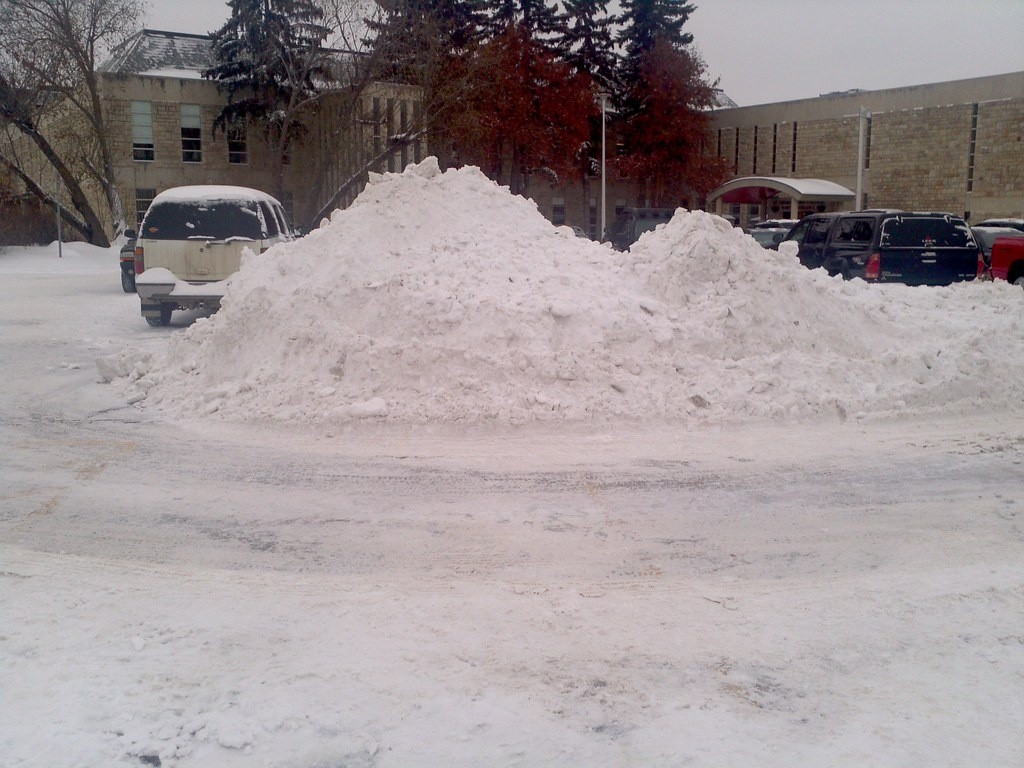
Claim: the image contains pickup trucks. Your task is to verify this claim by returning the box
[766,209,985,286]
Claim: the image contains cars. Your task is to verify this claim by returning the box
[973,218,1024,233]
[968,224,1024,288]
[749,218,800,249]
[120,227,139,294]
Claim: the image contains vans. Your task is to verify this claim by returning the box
[135,183,302,325]
